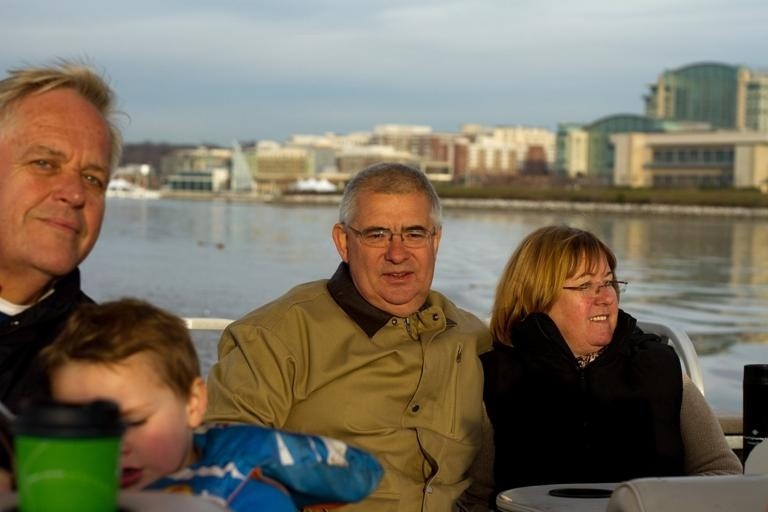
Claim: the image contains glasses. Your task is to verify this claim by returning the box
[562,280,628,297]
[342,221,441,248]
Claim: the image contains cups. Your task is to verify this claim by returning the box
[13,394,120,512]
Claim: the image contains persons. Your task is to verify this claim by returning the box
[41,296,384,512]
[484,225,742,485]
[0,51,132,494]
[206,163,498,512]
